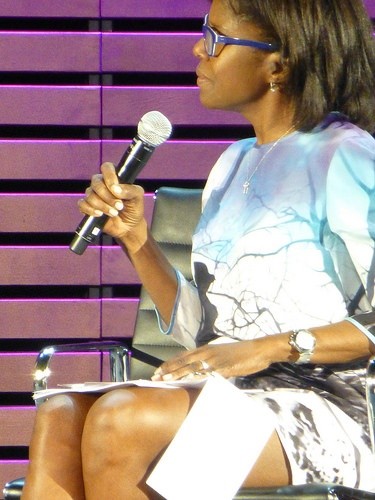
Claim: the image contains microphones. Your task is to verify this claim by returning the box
[71,111,173,257]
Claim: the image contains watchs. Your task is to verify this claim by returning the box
[289,328,318,365]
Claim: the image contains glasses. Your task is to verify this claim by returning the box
[202,12,279,56]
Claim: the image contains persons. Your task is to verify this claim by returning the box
[16,0,375,499]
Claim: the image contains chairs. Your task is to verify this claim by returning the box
[235,485,375,500]
[34,188,205,408]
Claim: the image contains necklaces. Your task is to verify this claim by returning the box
[241,115,300,193]
